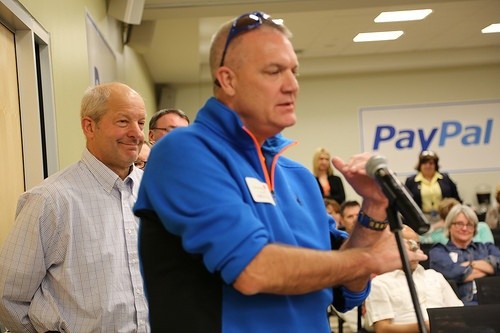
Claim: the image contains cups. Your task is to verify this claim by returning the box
[476,184,492,205]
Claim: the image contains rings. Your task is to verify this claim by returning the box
[408,240,418,251]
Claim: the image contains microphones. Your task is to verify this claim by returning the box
[365,155,431,235]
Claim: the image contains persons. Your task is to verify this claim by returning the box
[429,205,500,307]
[404,148,461,224]
[131,11,427,333]
[425,198,494,245]
[312,148,345,205]
[332,201,361,333]
[324,199,345,230]
[148,109,190,146]
[135,142,152,170]
[0,82,151,333]
[366,225,465,333]
[486,191,500,248]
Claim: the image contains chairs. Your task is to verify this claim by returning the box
[419,244,500,333]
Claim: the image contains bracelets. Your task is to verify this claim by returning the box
[470,260,472,267]
[358,209,388,231]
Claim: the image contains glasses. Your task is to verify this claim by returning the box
[150,126,176,133]
[214,11,271,87]
[420,151,437,160]
[451,221,475,231]
